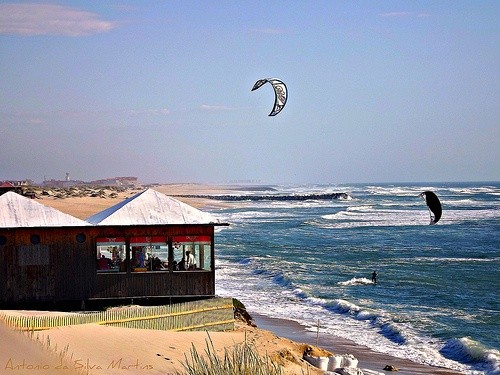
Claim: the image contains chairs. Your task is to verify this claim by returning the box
[97,260,189,273]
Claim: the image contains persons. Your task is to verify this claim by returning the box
[176,257,188,271]
[101,254,106,260]
[184,250,197,271]
[371,270,378,284]
